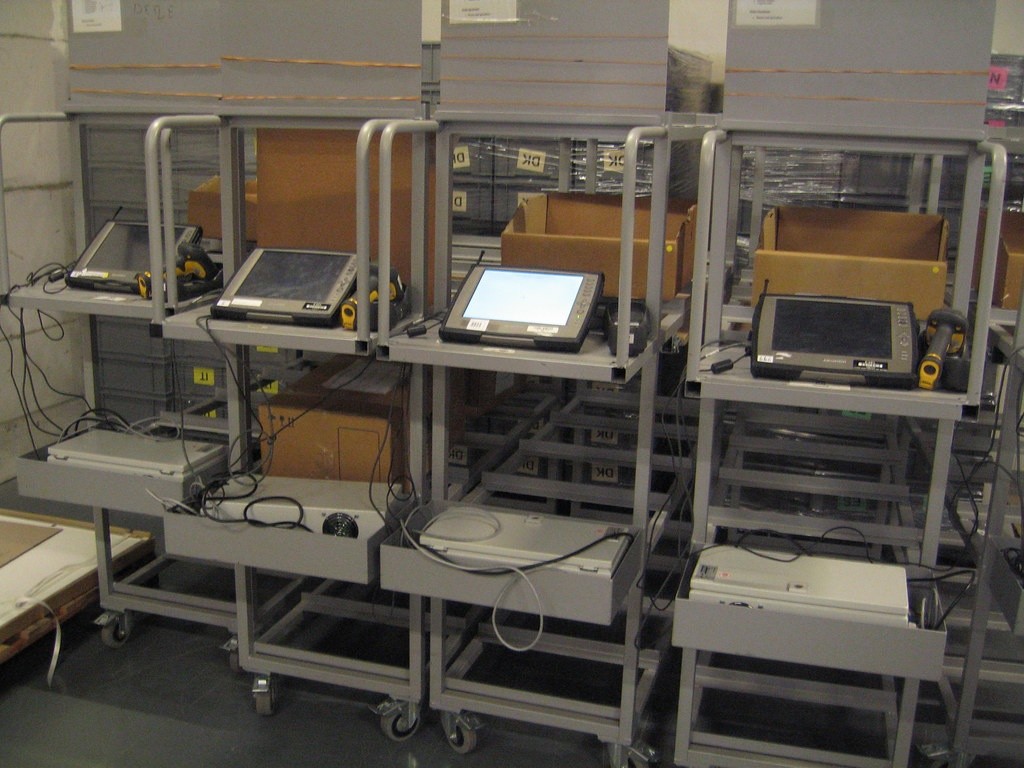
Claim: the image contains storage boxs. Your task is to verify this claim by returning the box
[255,127,439,316]
[188,175,259,244]
[257,354,469,496]
[970,207,1024,312]
[464,368,529,423]
[499,189,700,301]
[750,204,950,323]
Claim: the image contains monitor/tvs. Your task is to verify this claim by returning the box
[65,218,203,295]
[439,263,606,353]
[211,245,359,328]
[751,292,921,389]
